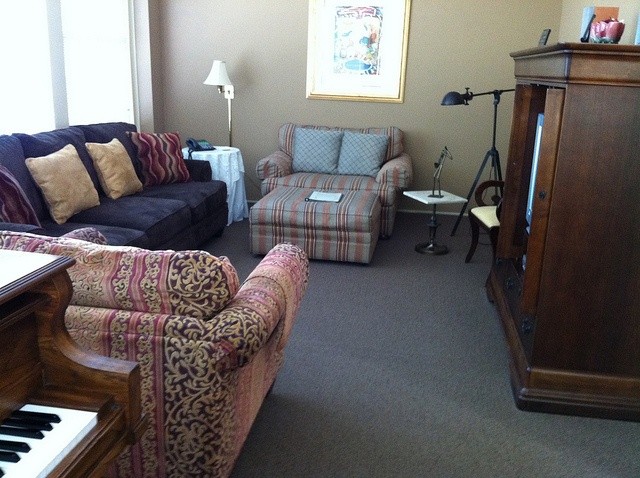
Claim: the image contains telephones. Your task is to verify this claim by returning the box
[186,137,215,160]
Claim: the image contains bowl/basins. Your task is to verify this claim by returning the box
[590,19,625,44]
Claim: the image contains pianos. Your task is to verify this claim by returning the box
[0,249,149,478]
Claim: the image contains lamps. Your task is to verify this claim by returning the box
[440,88,515,234]
[203,59,231,94]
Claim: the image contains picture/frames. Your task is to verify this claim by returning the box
[305,0,414,103]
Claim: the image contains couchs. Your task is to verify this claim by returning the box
[1,121,229,252]
[249,120,414,234]
[0,225,310,477]
[248,187,382,264]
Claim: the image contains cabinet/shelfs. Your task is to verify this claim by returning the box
[484,41,639,425]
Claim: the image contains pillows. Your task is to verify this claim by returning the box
[84,138,145,202]
[291,128,343,175]
[23,143,102,225]
[125,128,190,186]
[339,130,390,179]
[1,166,42,229]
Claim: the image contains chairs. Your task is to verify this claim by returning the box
[465,179,505,266]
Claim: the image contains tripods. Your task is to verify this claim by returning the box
[451,88,518,237]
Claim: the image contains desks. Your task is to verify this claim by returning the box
[402,189,469,256]
[181,145,249,226]
